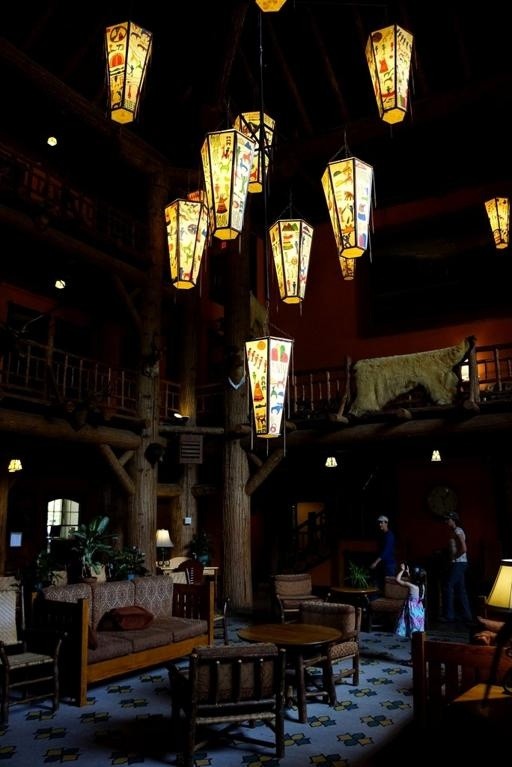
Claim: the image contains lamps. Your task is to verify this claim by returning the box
[154,529,173,568]
[486,558,512,614]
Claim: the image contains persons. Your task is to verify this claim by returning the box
[395,559,429,666]
[441,511,474,624]
[369,515,397,576]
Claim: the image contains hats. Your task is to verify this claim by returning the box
[378,516,388,522]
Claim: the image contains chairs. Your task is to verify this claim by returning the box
[264,572,324,624]
[411,594,512,736]
[165,642,287,767]
[369,576,409,634]
[288,600,361,707]
[0,576,68,729]
[171,558,229,646]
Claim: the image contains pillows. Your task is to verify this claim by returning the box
[96,605,154,631]
[89,625,98,649]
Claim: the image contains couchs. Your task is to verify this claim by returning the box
[35,569,215,707]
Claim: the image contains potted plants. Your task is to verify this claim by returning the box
[344,562,371,607]
[35,516,149,587]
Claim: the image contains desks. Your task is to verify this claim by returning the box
[237,623,341,724]
[332,585,378,607]
[157,565,219,612]
